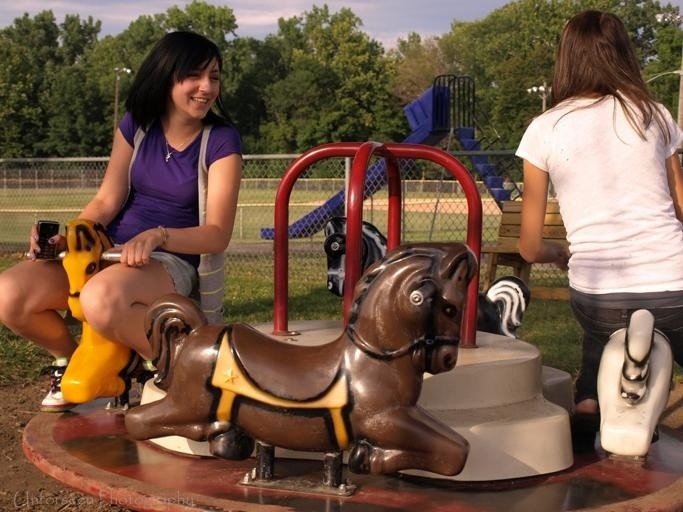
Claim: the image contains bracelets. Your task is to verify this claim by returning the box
[157,225,168,251]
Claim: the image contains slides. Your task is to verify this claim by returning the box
[260,87,451,240]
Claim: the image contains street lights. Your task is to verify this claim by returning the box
[527,80,552,114]
[111,65,132,138]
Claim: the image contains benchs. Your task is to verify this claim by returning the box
[479,199,575,296]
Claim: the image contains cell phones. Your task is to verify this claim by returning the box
[34,220,60,258]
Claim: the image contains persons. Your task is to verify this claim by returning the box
[515,11,683,435]
[0,31,243,413]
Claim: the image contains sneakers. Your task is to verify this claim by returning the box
[37,353,80,412]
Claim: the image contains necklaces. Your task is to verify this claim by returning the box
[163,128,192,163]
